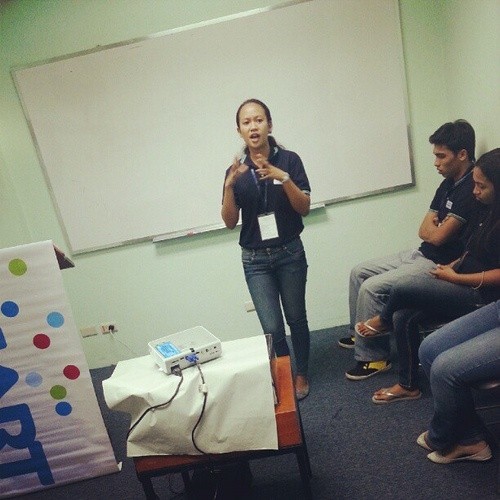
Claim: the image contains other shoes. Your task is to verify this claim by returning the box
[418,430,435,451]
[427,445,492,465]
[296,380,310,401]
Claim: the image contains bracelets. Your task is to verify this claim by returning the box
[281,172,289,183]
[472,271,484,290]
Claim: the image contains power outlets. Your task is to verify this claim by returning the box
[101,323,117,333]
[245,301,255,311]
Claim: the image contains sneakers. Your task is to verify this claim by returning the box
[339,337,356,349]
[345,357,392,380]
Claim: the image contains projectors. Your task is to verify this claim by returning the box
[148,326,222,374]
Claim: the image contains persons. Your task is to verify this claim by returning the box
[354,146,500,403]
[339,120,477,380]
[221,99,311,402]
[416,301,500,463]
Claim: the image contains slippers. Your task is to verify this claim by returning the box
[355,319,392,337]
[371,386,423,405]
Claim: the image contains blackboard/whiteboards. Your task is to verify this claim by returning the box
[9,0,417,258]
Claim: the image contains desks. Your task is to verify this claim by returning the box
[135,355,314,500]
